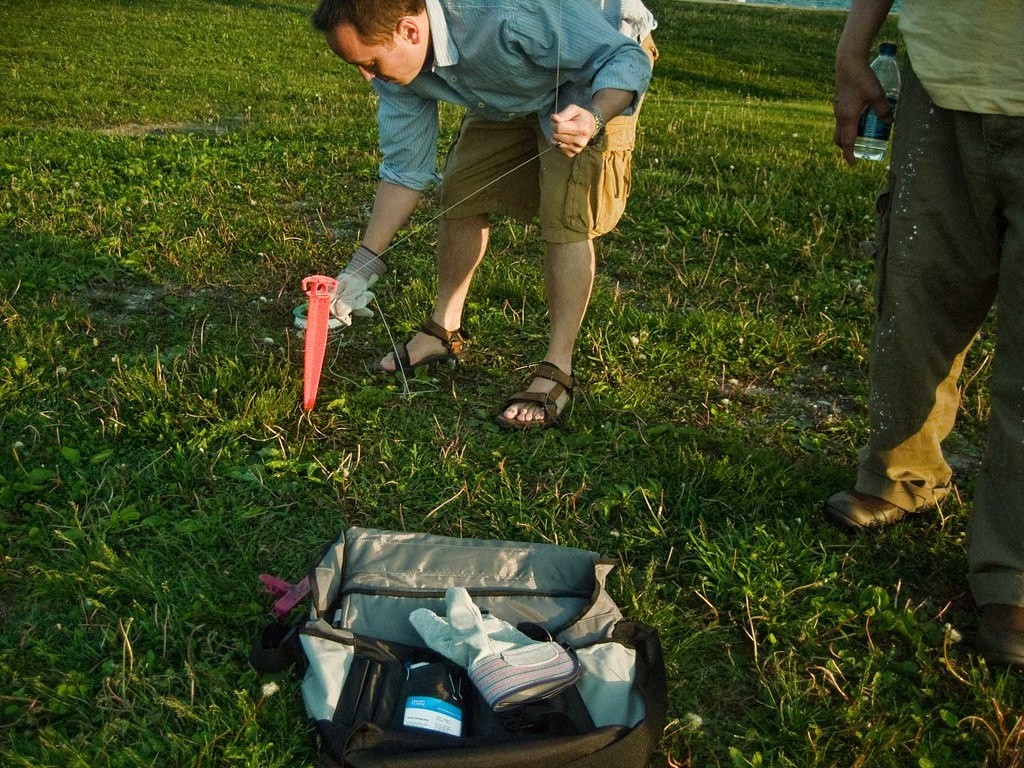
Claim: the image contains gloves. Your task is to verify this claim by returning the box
[409,585,582,711]
[328,245,389,327]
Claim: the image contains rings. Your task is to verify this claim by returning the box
[554,141,562,148]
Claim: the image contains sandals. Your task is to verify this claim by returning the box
[493,361,575,431]
[374,318,469,374]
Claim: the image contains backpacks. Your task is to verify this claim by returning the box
[249,525,671,768]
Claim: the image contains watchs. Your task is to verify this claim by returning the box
[585,107,605,146]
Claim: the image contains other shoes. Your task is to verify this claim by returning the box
[822,487,908,535]
[974,602,1024,667]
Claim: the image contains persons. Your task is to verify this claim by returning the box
[823,0,1024,663]
[311,0,659,429]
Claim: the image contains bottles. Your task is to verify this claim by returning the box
[853,42,901,160]
